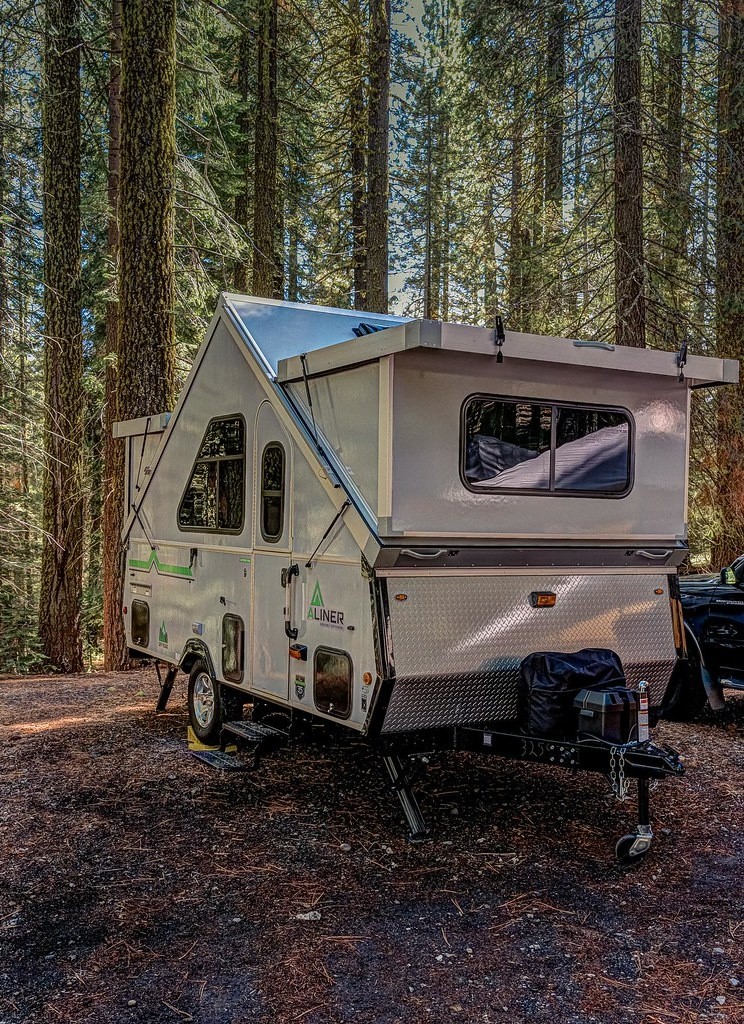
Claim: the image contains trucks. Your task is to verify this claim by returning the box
[113,291,740,867]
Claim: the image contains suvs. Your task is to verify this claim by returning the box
[659,553,744,718]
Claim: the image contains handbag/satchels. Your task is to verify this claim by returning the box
[517,647,627,737]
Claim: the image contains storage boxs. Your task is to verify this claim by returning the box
[574,685,640,746]
